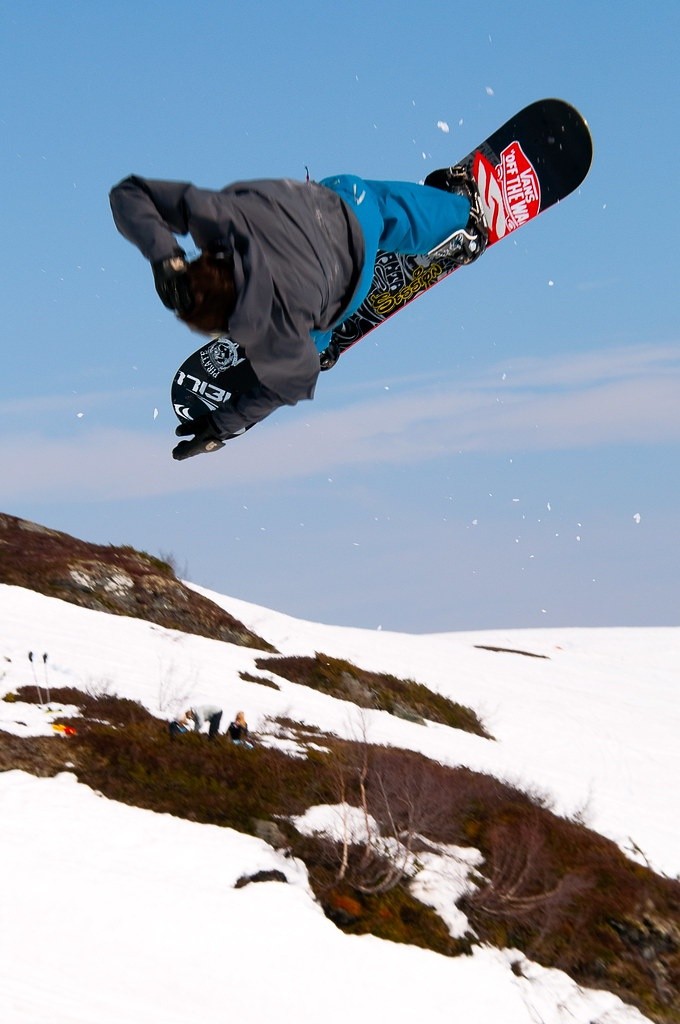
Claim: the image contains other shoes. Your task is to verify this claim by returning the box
[445,164,487,262]
[318,336,337,370]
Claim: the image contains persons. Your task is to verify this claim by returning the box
[168,707,254,750]
[107,167,488,461]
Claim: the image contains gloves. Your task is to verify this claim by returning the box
[172,413,226,461]
[151,254,191,311]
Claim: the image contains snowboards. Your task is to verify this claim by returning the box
[168,95,595,443]
